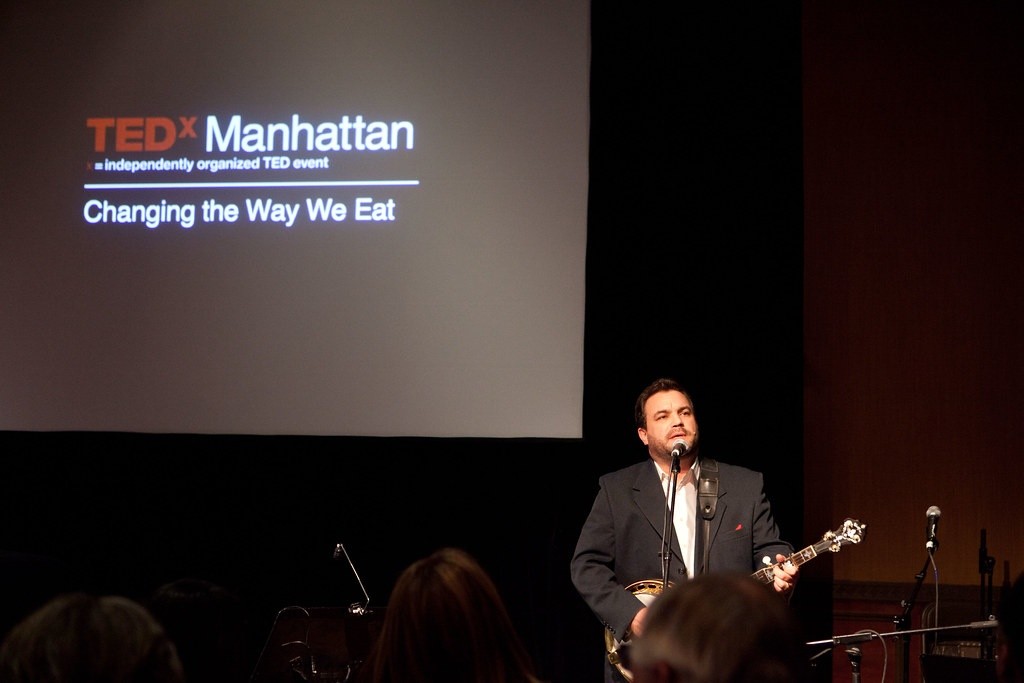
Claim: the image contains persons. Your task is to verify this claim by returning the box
[632,573,794,683]
[150,578,249,683]
[995,572,1024,683]
[0,592,185,683]
[356,548,541,683]
[571,380,800,683]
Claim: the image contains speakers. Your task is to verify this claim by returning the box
[925,633,984,659]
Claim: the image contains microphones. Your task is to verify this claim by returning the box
[926,506,942,551]
[671,438,689,457]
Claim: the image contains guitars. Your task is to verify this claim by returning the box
[604,517,868,683]
